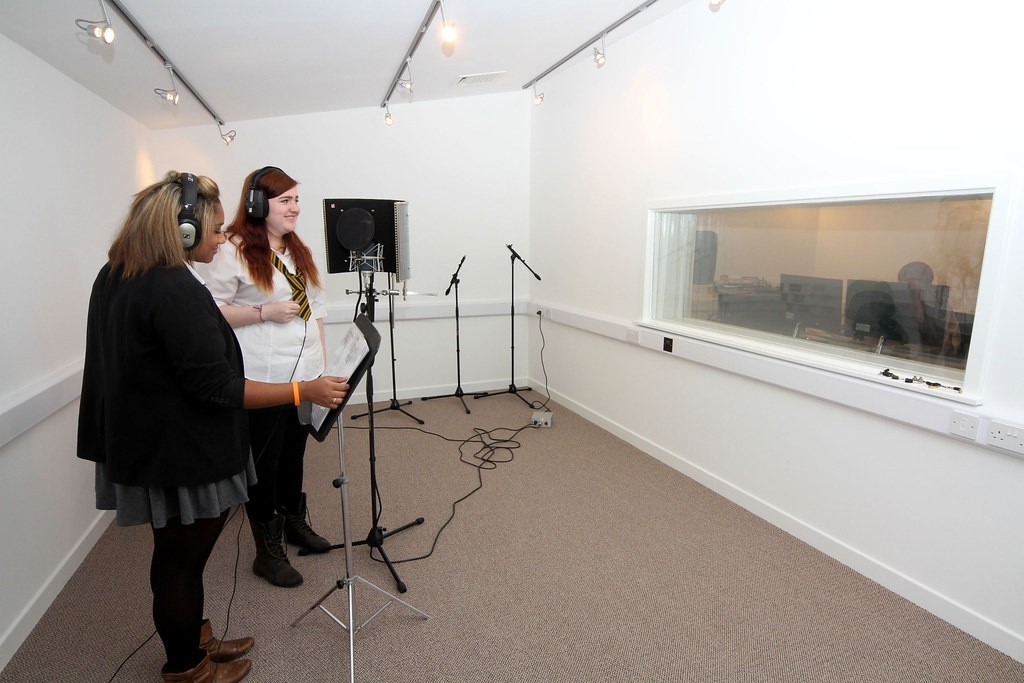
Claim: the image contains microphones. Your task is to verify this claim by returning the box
[362,242,375,290]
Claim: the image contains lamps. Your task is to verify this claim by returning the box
[214,116,237,146]
[153,62,180,105]
[395,56,414,93]
[75,0,116,44]
[384,104,394,126]
[594,34,606,66]
[532,82,545,106]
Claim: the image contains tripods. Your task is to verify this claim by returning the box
[421,256,488,414]
[474,244,541,409]
[290,273,432,683]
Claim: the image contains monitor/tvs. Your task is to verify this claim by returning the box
[846,279,949,349]
[780,275,843,334]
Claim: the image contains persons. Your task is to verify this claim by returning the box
[867,261,961,353]
[76,172,351,683]
[205,165,332,587]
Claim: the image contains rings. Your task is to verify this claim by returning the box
[333,398,336,403]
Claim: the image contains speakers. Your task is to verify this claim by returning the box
[693,231,718,284]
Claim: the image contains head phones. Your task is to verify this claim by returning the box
[245,166,284,223]
[177,172,201,250]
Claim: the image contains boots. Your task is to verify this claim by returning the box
[282,492,333,554]
[163,651,252,682]
[199,618,256,658]
[253,519,305,587]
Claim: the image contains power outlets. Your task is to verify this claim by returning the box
[986,420,1024,455]
[663,337,674,353]
[535,306,545,318]
[625,328,641,343]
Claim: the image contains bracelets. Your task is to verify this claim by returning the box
[254,304,265,323]
[293,382,302,406]
